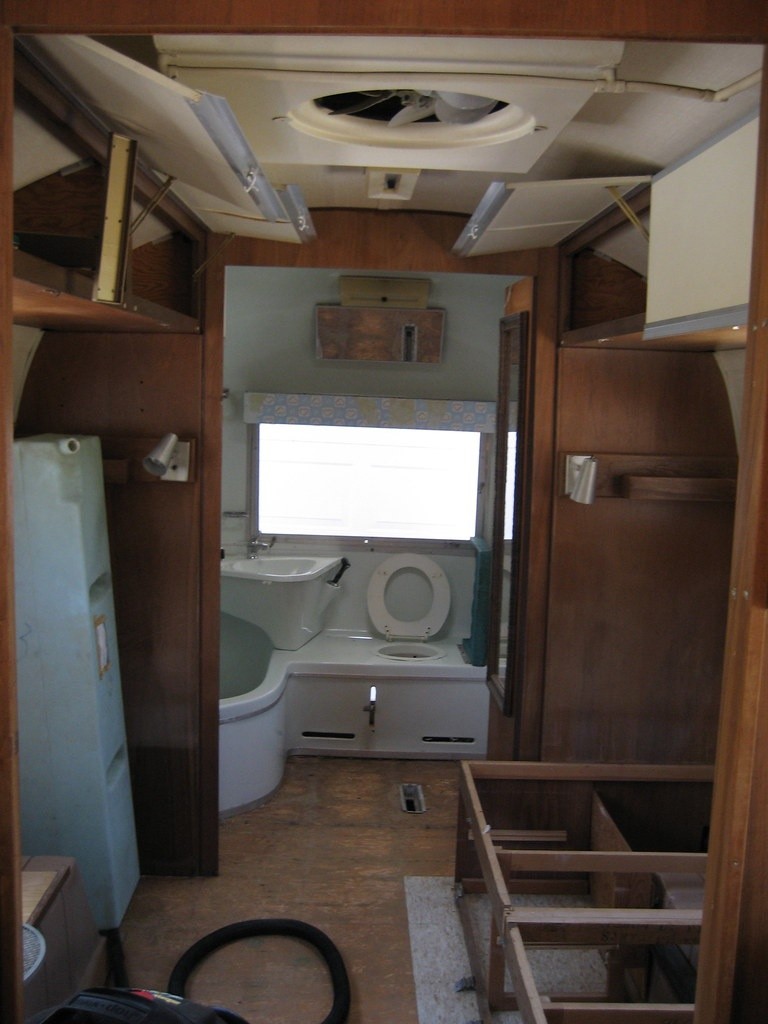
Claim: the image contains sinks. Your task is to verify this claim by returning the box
[220,551,345,652]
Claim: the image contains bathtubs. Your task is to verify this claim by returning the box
[219,612,289,822]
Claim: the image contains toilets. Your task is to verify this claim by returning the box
[367,551,451,661]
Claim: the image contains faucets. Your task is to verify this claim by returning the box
[246,531,268,560]
